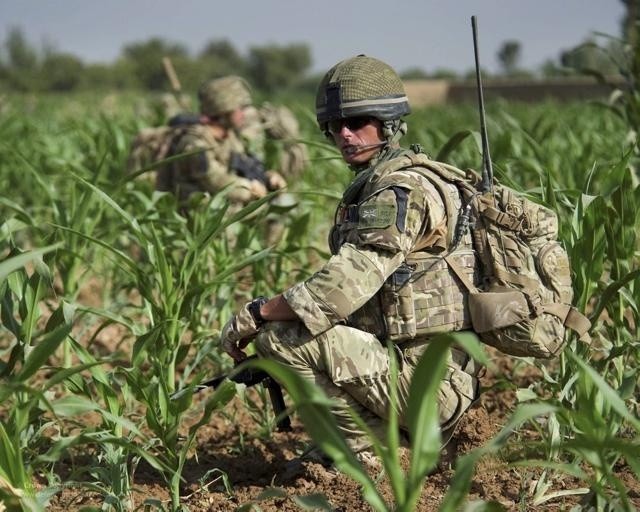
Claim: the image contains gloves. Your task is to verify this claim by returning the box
[264,168,288,191]
[221,301,262,362]
[251,177,268,200]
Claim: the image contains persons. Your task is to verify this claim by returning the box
[239,100,312,175]
[221,53,576,476]
[127,74,297,250]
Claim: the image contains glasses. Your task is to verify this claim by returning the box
[324,115,378,136]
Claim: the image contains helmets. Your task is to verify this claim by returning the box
[314,52,413,128]
[197,73,254,120]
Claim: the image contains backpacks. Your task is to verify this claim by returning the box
[125,125,239,184]
[364,153,594,360]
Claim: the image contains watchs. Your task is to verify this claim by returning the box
[251,295,270,321]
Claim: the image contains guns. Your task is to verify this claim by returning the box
[198,355,294,431]
[225,151,276,206]
